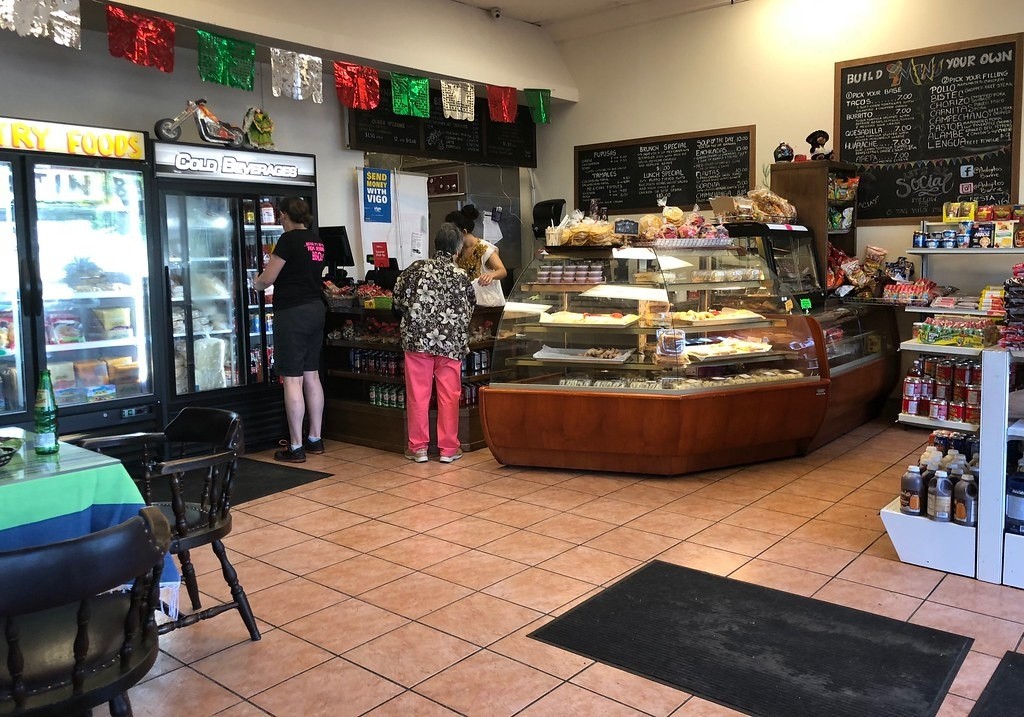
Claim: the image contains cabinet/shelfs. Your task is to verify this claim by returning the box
[319,162,1024,591]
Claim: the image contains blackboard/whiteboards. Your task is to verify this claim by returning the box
[574,124,755,215]
[833,29,1024,225]
[349,79,537,168]
[615,219,640,236]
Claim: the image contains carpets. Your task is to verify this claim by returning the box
[970,650,1024,717]
[133,458,335,509]
[527,557,972,717]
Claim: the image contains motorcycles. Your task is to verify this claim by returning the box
[152,94,248,149]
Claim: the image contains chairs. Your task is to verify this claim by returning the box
[0,506,172,717]
[96,409,261,640]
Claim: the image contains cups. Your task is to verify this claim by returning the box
[925,220,972,249]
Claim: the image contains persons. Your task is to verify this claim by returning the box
[390,223,478,464]
[444,205,508,336]
[249,196,330,463]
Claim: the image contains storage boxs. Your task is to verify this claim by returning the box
[47,361,76,389]
[994,221,1019,248]
[71,359,111,386]
[92,308,130,331]
[100,355,132,387]
[970,222,994,249]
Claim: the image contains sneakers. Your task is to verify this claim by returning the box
[302,439,324,454]
[272,439,306,463]
[404,446,429,462]
[439,448,463,463]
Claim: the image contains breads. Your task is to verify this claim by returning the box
[690,268,765,282]
[558,312,803,390]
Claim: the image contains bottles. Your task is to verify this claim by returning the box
[900,447,979,527]
[251,343,276,383]
[246,271,259,305]
[248,309,274,334]
[34,370,60,455]
[913,231,930,248]
[245,234,281,269]
[242,198,276,225]
[907,360,922,379]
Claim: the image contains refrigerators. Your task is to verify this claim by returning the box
[151,139,319,464]
[0,115,153,471]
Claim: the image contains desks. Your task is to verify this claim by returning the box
[0,425,183,618]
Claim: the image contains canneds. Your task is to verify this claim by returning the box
[912,230,930,248]
[902,358,981,421]
[349,348,407,410]
[461,348,490,407]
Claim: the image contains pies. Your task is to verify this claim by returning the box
[561,222,616,246]
[638,214,665,240]
[663,206,684,228]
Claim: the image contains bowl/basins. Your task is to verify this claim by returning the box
[536,264,602,283]
[0,437,24,467]
